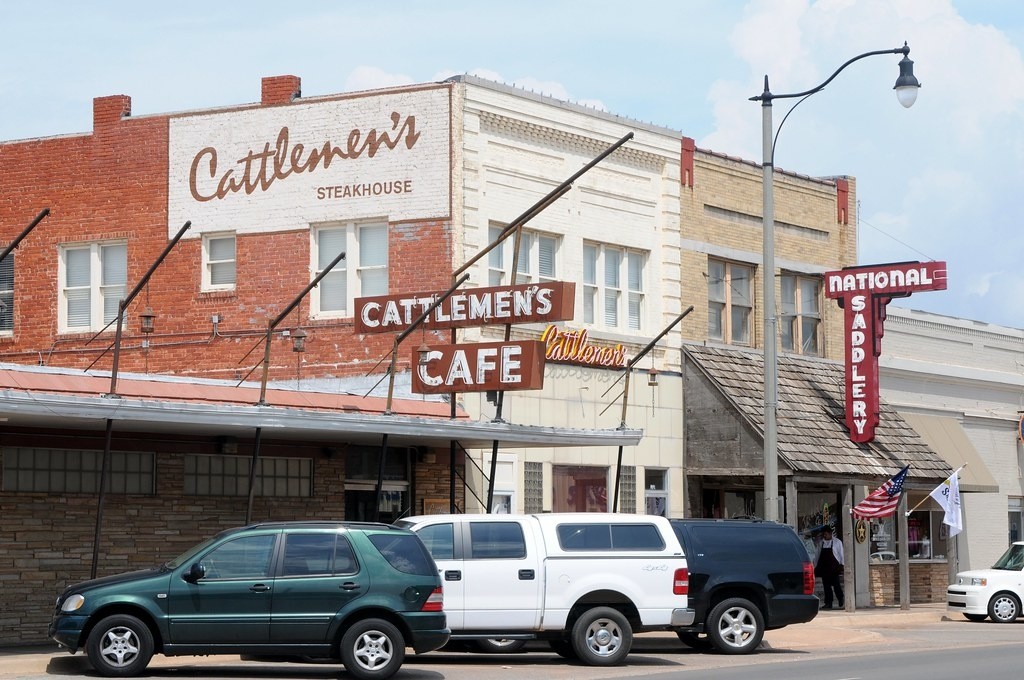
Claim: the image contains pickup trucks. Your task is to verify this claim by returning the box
[354,510,697,667]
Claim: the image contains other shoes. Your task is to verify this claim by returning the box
[839,597,844,606]
[822,604,832,608]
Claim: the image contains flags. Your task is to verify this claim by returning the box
[929,467,963,538]
[853,464,910,522]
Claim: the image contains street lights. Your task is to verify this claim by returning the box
[747,41,924,527]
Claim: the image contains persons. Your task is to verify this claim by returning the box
[813,525,844,609]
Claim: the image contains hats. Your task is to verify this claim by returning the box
[822,525,833,533]
[803,528,813,536]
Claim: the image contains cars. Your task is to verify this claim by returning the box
[944,541,1024,620]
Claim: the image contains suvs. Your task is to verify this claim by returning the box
[561,513,822,655]
[48,519,451,680]
